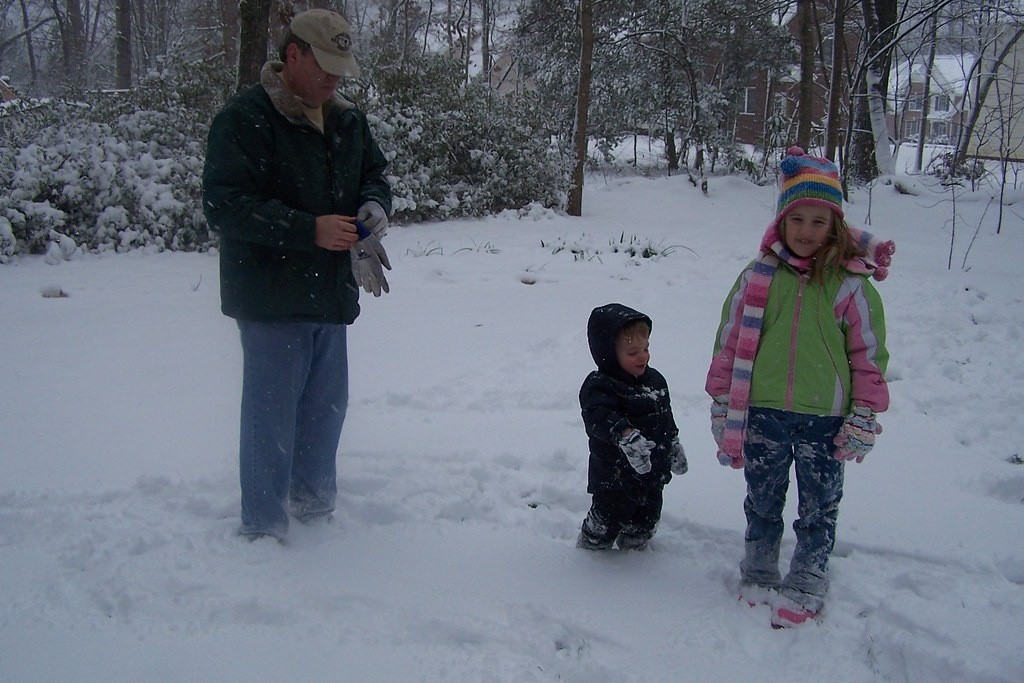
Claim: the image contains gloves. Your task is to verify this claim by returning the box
[833,405,884,464]
[357,200,387,239]
[618,428,657,474]
[349,220,392,297]
[668,439,690,475]
[710,403,746,468]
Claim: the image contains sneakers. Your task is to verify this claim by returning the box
[738,584,772,606]
[773,600,816,626]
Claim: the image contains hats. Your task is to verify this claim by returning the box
[289,8,361,77]
[775,146,844,224]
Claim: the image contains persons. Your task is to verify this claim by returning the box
[578,303,688,552]
[705,146,895,629]
[201,7,391,549]
